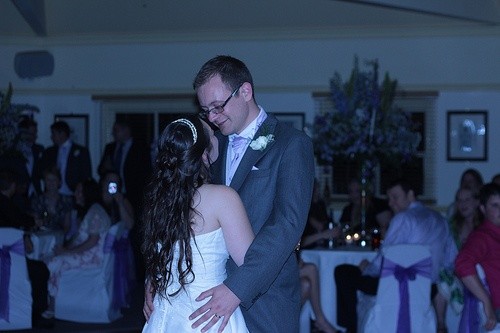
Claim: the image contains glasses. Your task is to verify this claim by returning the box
[198,85,241,119]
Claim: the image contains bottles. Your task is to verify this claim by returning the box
[326,208,337,251]
[372,228,380,252]
[321,177,330,206]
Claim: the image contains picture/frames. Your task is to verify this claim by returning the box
[55,113,89,152]
[447,110,488,162]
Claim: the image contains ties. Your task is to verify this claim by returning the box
[112,142,124,176]
[226,136,250,186]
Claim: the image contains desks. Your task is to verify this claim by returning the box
[302,246,380,330]
[29,231,65,260]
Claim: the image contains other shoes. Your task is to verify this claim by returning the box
[41,310,54,318]
[312,320,342,333]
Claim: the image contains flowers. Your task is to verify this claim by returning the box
[249,122,275,152]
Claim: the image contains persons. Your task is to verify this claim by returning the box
[142,56,314,333]
[0,112,154,318]
[142,114,255,333]
[296,169,500,333]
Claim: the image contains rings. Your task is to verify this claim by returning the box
[215,313,221,318]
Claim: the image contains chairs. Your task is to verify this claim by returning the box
[0,222,500,333]
